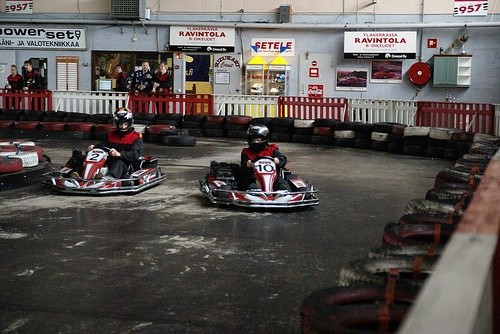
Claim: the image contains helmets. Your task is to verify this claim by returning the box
[246,123,270,151]
[113,107,134,134]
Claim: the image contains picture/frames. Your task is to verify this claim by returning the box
[369,60,403,84]
[335,67,369,92]
[432,54,471,88]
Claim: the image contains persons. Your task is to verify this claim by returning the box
[113,60,173,113]
[70,107,144,186]
[22,60,45,111]
[238,123,292,192]
[5,64,24,110]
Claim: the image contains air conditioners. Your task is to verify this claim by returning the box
[108,0,150,20]
